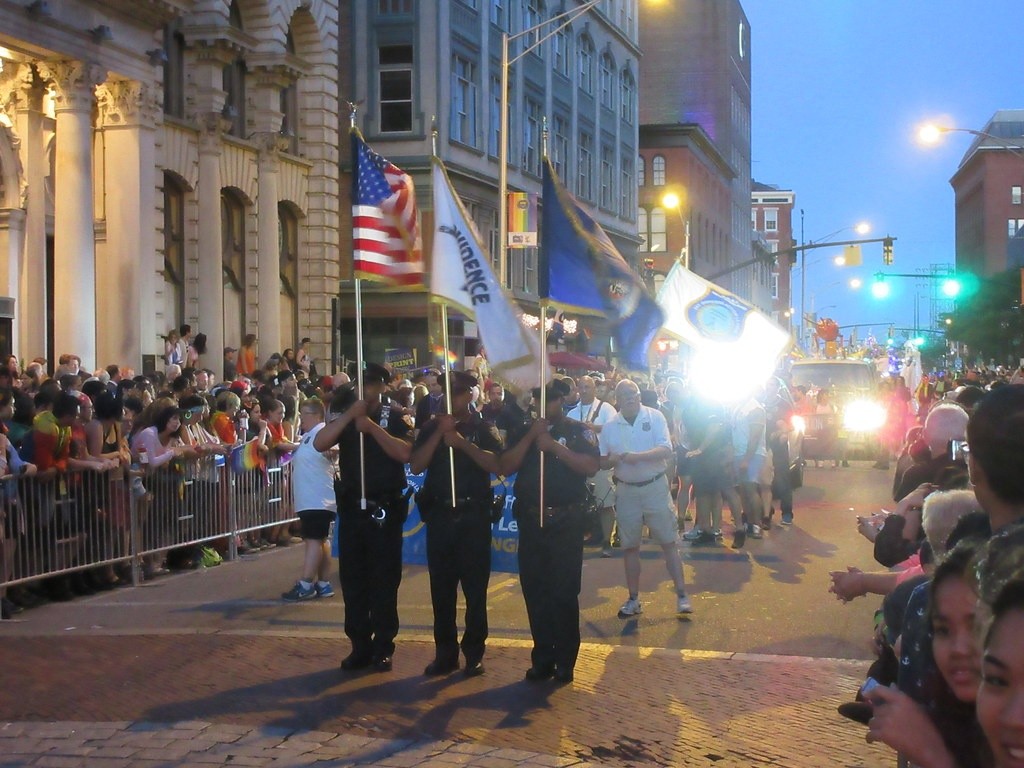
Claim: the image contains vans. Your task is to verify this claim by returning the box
[789,362,885,464]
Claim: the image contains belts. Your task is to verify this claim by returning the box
[617,471,664,487]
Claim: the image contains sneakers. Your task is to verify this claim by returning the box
[280,580,317,602]
[315,582,334,597]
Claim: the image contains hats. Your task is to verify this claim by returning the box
[472,339,485,356]
[532,377,571,399]
[347,361,391,386]
[398,379,413,389]
[437,370,478,393]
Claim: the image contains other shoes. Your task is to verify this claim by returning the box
[677,597,692,613]
[465,660,485,676]
[556,666,574,683]
[618,598,641,615]
[0,527,310,619]
[525,663,555,678]
[424,656,460,676]
[584,505,793,559]
[341,652,393,671]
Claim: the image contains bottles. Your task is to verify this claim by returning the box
[139,444,149,467]
[240,409,248,431]
[215,453,226,467]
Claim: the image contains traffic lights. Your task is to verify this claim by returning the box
[917,333,922,344]
[942,280,959,296]
[887,332,894,345]
[872,279,887,295]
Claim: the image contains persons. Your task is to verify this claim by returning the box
[563,367,815,553]
[829,366,1024,768]
[178,324,192,371]
[379,362,445,428]
[469,342,536,449]
[0,337,361,619]
[600,379,694,616]
[165,330,183,366]
[815,388,836,468]
[189,334,207,369]
[410,371,502,674]
[236,334,256,376]
[311,361,413,669]
[502,378,600,681]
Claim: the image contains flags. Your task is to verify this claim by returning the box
[541,155,663,366]
[430,156,553,391]
[655,262,793,396]
[813,329,921,396]
[350,125,423,291]
[231,439,259,475]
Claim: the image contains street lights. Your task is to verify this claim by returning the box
[500,0,668,290]
[663,191,688,269]
[788,256,846,334]
[810,278,863,333]
[801,224,870,344]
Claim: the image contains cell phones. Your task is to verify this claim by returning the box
[948,439,971,462]
[863,677,884,705]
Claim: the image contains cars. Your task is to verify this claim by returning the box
[772,377,804,487]
